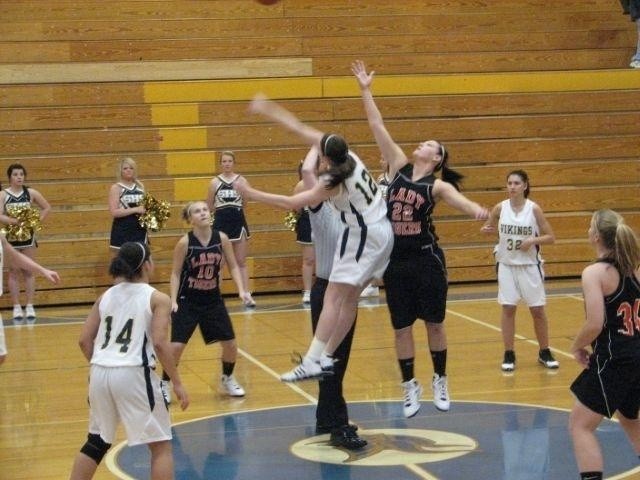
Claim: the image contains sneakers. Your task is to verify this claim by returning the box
[303,294,310,303]
[281,359,335,381]
[538,349,559,368]
[13,306,36,318]
[160,379,170,404]
[501,350,515,371]
[331,425,366,450]
[432,373,449,411]
[401,378,421,417]
[220,375,244,396]
[359,284,379,297]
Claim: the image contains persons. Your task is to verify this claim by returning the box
[505,370,557,479]
[171,413,241,480]
[158,201,247,404]
[361,155,389,298]
[109,157,171,284]
[293,161,322,304]
[205,151,256,306]
[0,230,60,367]
[350,61,492,420]
[302,140,369,449]
[67,242,190,479]
[234,95,394,383]
[0,164,51,319]
[567,210,640,480]
[620,0,640,70]
[480,169,561,369]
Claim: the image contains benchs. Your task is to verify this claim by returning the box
[0,0,640,306]
